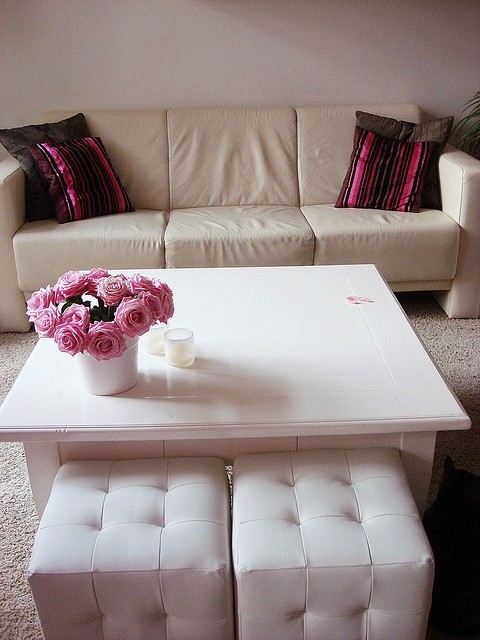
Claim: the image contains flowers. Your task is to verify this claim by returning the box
[27,268,175,361]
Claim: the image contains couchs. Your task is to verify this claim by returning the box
[0,104,480,333]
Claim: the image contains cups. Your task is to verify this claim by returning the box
[142,320,166,356]
[165,328,196,368]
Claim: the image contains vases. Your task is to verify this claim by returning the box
[78,332,138,398]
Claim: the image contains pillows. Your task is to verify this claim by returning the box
[333,125,437,213]
[0,113,103,222]
[28,135,133,224]
[350,111,454,210]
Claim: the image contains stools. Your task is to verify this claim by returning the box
[29,458,234,639]
[229,447,433,640]
